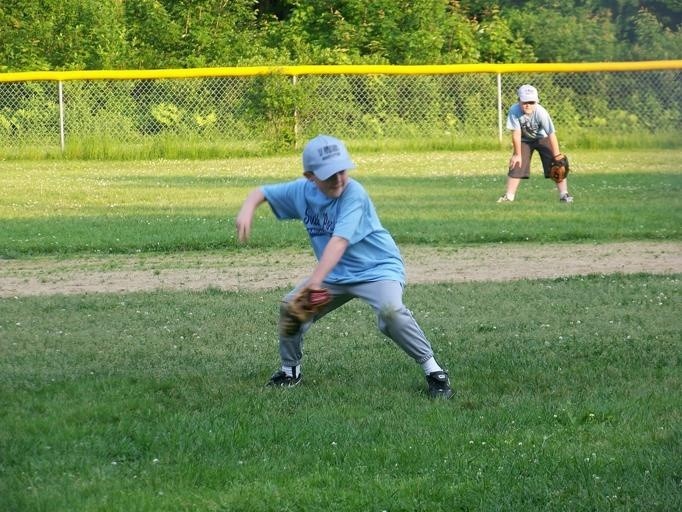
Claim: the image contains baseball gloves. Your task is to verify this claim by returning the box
[548,155,569,183]
[281,284,330,337]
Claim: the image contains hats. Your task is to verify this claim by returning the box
[518,84,539,104]
[303,135,357,182]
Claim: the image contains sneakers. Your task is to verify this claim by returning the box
[497,195,513,202]
[427,368,454,401]
[561,193,573,203]
[266,372,303,391]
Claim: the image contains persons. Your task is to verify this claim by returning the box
[495,85,574,205]
[235,133,454,402]
